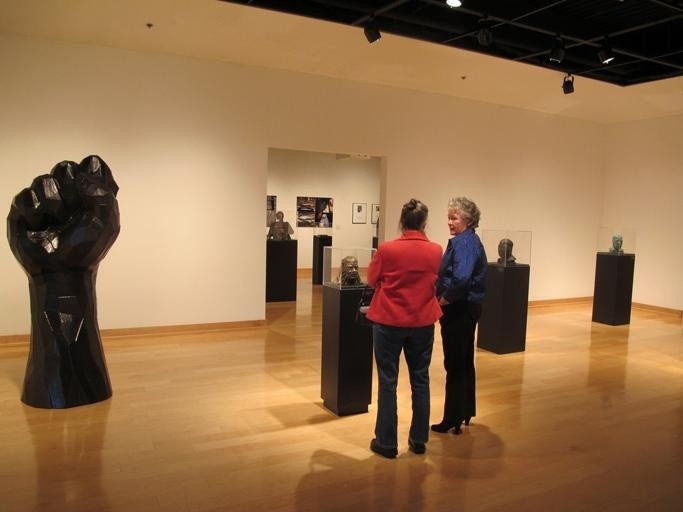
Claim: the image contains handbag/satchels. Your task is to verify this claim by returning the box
[356,302,373,326]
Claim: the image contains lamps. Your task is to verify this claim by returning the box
[363,18,381,44]
[549,36,615,95]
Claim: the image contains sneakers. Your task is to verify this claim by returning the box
[371,439,397,457]
[408,436,425,454]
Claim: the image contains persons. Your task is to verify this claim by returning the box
[497,239,517,264]
[430,195,487,434]
[364,198,444,459]
[335,255,362,285]
[266,210,294,240]
[609,233,624,257]
[5,153,120,410]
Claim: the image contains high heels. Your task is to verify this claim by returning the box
[431,416,471,434]
